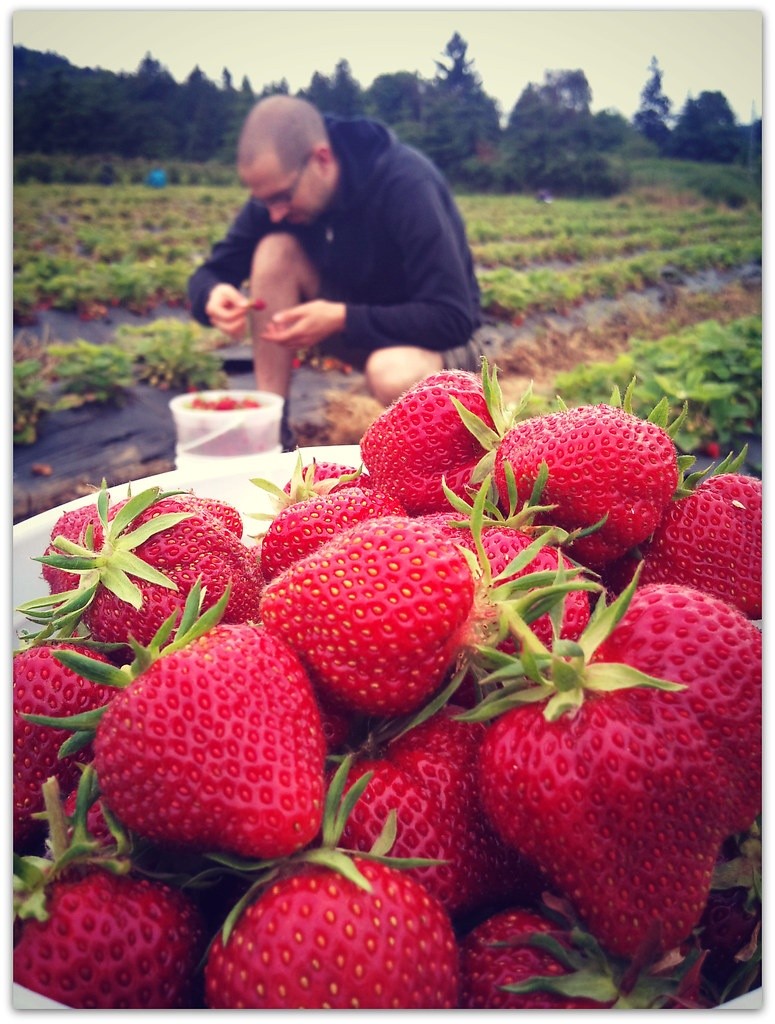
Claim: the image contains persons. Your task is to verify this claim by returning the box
[188,95,482,455]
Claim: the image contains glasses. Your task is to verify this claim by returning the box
[250,151,314,208]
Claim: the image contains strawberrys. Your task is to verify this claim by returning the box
[247,298,267,311]
[14,355,763,1011]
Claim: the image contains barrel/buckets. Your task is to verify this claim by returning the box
[12,444,775,1012]
[167,388,285,468]
